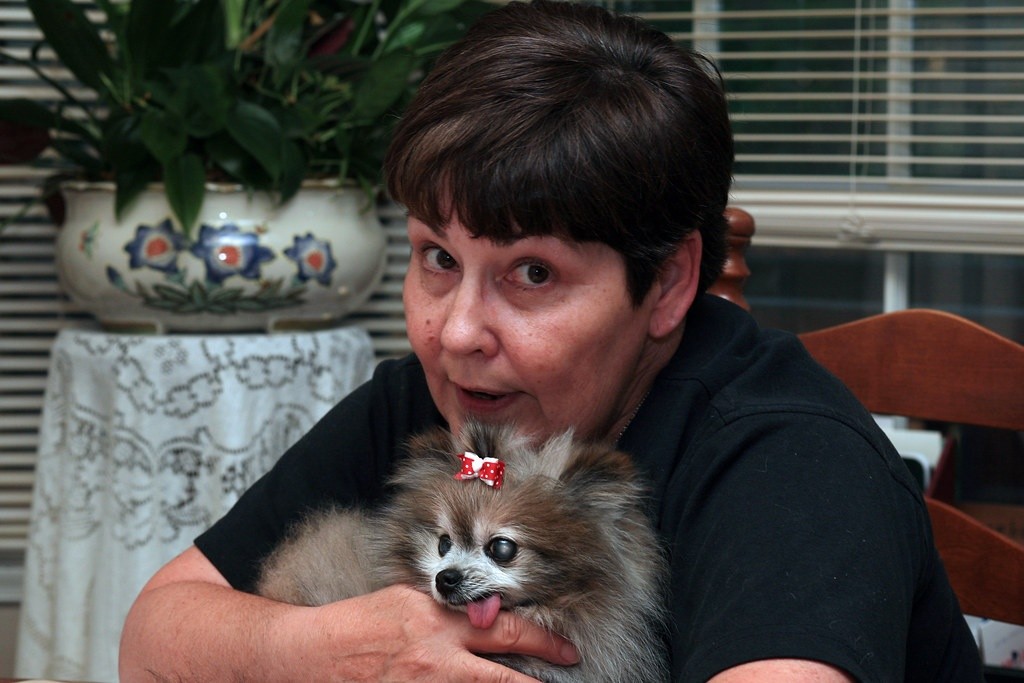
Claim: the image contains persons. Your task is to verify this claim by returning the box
[114,0,986,683]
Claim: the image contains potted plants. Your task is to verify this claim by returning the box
[0,0,486,339]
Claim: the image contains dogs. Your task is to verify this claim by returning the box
[256,423,676,681]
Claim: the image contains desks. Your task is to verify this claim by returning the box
[15,322,380,683]
